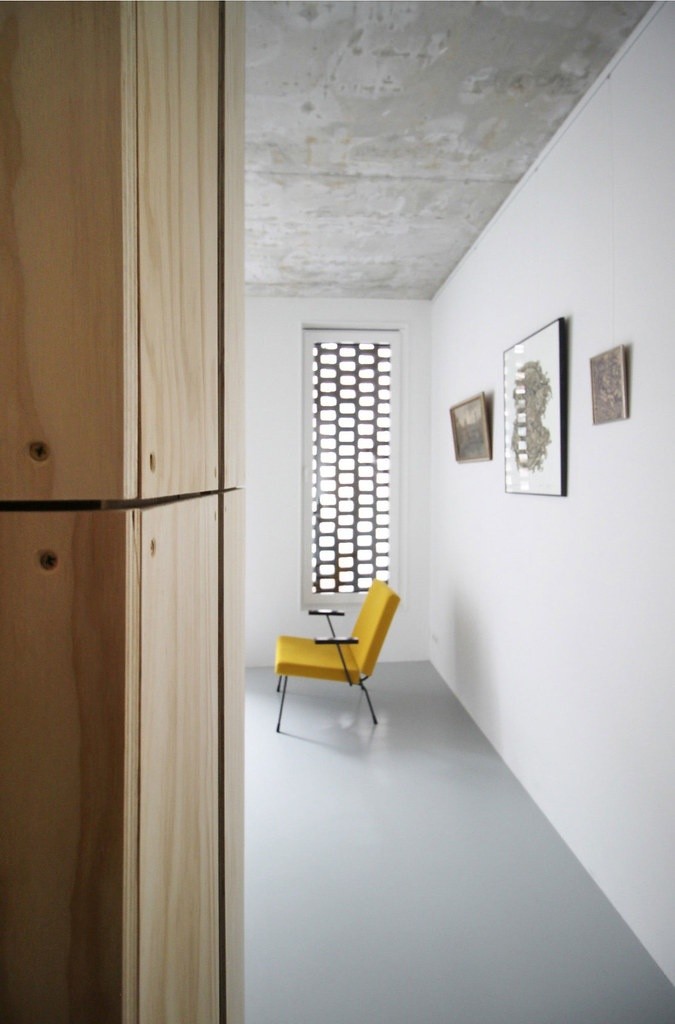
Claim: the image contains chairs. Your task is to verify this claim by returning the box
[276,580,401,733]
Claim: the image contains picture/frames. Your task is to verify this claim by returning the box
[590,344,628,425]
[503,318,568,496]
[449,391,491,464]
